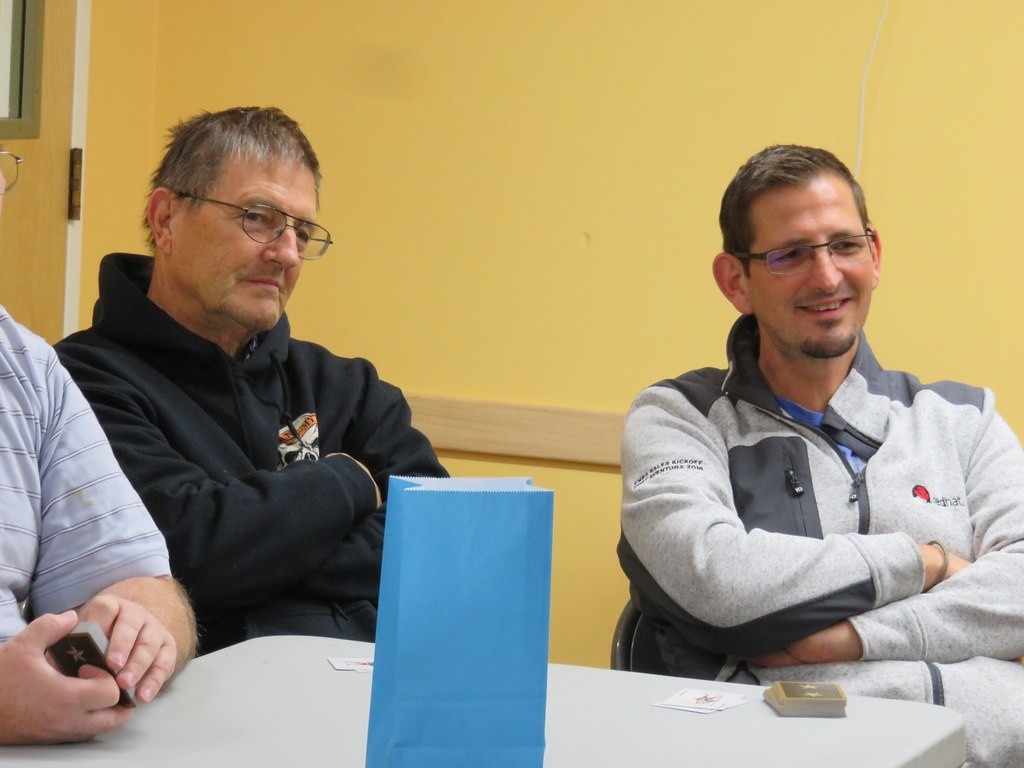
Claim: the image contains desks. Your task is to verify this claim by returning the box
[0,635,965,768]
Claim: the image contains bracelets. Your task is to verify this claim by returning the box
[926,539,949,586]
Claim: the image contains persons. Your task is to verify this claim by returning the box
[52,107,451,659]
[0,150,198,746]
[616,146,1024,768]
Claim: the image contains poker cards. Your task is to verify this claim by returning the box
[46,618,138,710]
[651,680,852,719]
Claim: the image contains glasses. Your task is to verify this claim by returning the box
[175,192,333,261]
[733,228,878,275]
